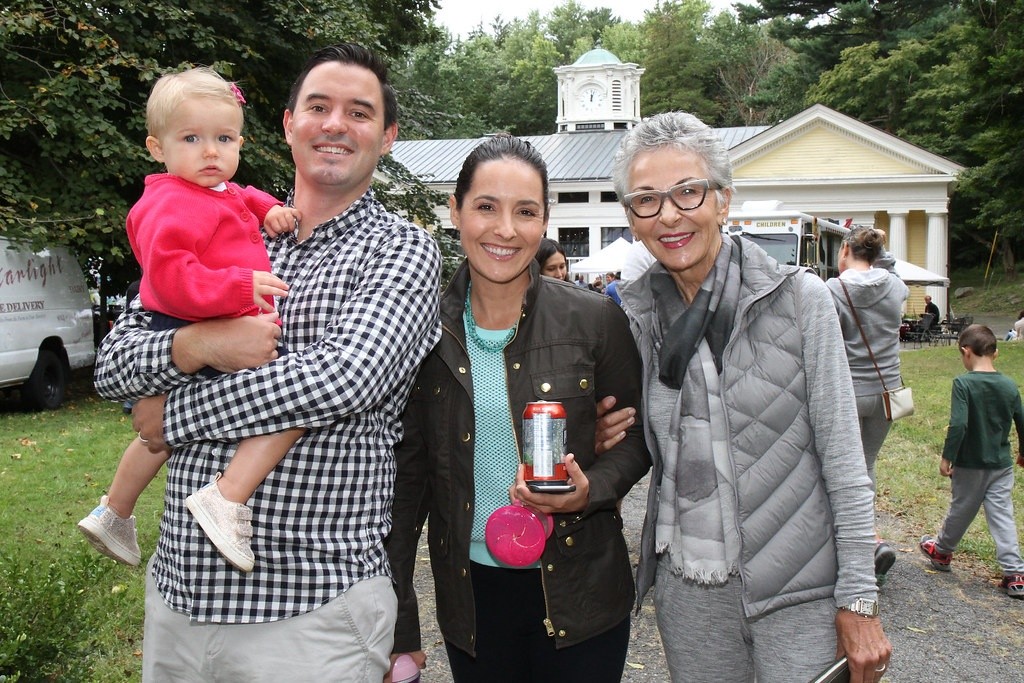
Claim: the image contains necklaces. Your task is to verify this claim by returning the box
[465,279,517,351]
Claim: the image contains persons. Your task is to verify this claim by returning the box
[920,324,1024,597]
[380,133,646,683]
[535,237,624,305]
[591,110,893,683]
[88,55,442,683]
[824,225,909,588]
[1006,310,1024,340]
[924,295,940,332]
[76,69,302,574]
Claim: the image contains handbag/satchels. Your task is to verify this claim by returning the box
[882,387,915,421]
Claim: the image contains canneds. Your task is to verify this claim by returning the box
[522,400,569,485]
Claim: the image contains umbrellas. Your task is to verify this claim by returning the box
[569,237,658,287]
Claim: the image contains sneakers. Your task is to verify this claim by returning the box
[919,535,953,571]
[184,471,255,571]
[996,574,1024,596]
[874,541,896,587]
[77,495,141,565]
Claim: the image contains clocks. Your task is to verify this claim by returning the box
[580,89,604,112]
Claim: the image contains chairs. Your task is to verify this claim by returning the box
[902,314,974,350]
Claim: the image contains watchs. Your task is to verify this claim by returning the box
[838,598,880,618]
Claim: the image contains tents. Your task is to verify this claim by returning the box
[893,255,951,346]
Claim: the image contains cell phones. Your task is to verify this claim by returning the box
[811,655,850,683]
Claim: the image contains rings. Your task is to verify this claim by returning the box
[138,432,148,445]
[874,664,886,672]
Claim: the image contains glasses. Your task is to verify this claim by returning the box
[623,179,721,218]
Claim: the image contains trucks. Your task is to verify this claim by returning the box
[722,200,853,284]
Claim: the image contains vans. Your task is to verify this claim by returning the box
[0,237,96,411]
